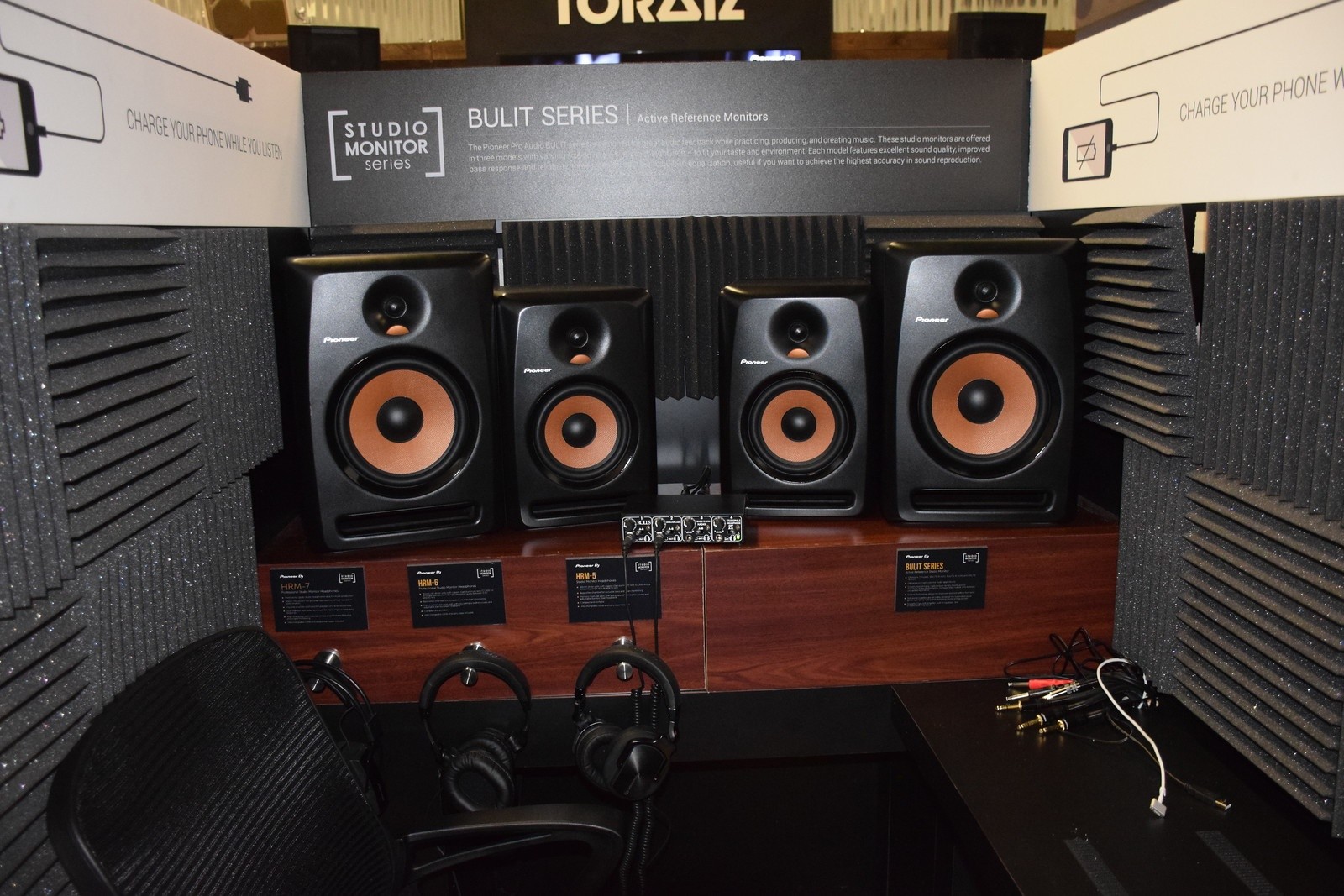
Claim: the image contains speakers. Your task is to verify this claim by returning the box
[286,25,381,72]
[493,284,657,528]
[871,237,1087,524]
[947,12,1047,60]
[280,250,501,551]
[718,279,881,518]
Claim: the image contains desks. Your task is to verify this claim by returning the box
[888,682,1344,896]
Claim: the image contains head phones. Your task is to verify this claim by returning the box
[293,659,388,817]
[572,645,682,803]
[419,649,532,813]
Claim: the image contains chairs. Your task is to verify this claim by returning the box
[49,626,624,896]
